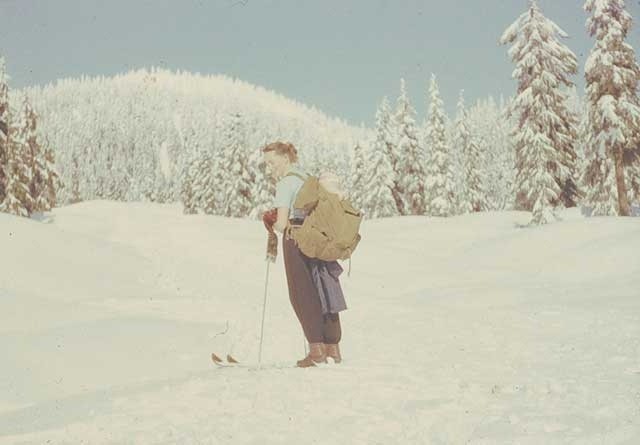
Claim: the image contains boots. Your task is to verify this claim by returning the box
[297,342,327,367]
[328,343,342,363]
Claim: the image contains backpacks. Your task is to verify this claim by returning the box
[286,171,365,262]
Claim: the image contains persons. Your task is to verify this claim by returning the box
[263,142,341,367]
